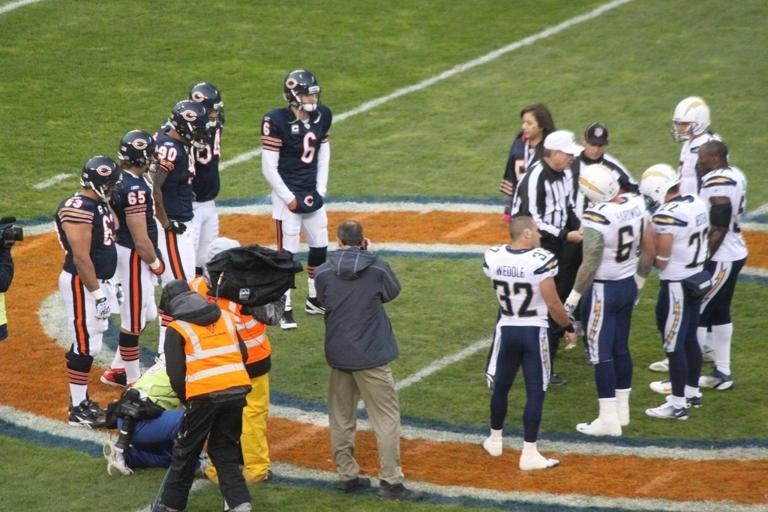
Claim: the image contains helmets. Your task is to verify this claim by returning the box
[638,163,683,214]
[670,95,711,142]
[80,81,222,208]
[283,69,321,114]
[578,163,621,203]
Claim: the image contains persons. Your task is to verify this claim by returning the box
[145,278,257,511]
[51,155,120,426]
[100,129,166,391]
[482,216,578,471]
[0,215,15,343]
[183,79,223,281]
[259,68,333,332]
[148,99,205,368]
[314,219,427,502]
[639,95,749,421]
[502,101,656,437]
[187,274,280,489]
[97,357,192,478]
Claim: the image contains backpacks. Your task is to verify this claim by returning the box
[208,243,304,308]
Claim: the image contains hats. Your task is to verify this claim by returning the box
[158,279,189,309]
[544,130,586,154]
[585,121,608,146]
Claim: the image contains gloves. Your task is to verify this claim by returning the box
[91,288,111,320]
[150,258,170,288]
[166,218,187,235]
[113,277,125,305]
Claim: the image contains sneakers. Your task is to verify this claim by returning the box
[68,389,108,415]
[378,480,423,501]
[304,294,326,315]
[279,309,297,329]
[100,365,127,388]
[481,357,733,471]
[338,477,371,492]
[68,400,106,430]
